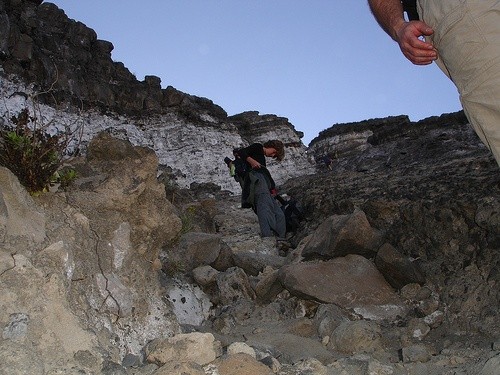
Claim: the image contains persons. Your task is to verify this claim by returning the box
[233,147,251,208]
[368,0,500,168]
[238,139,285,237]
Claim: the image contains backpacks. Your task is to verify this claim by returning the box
[223,145,247,182]
